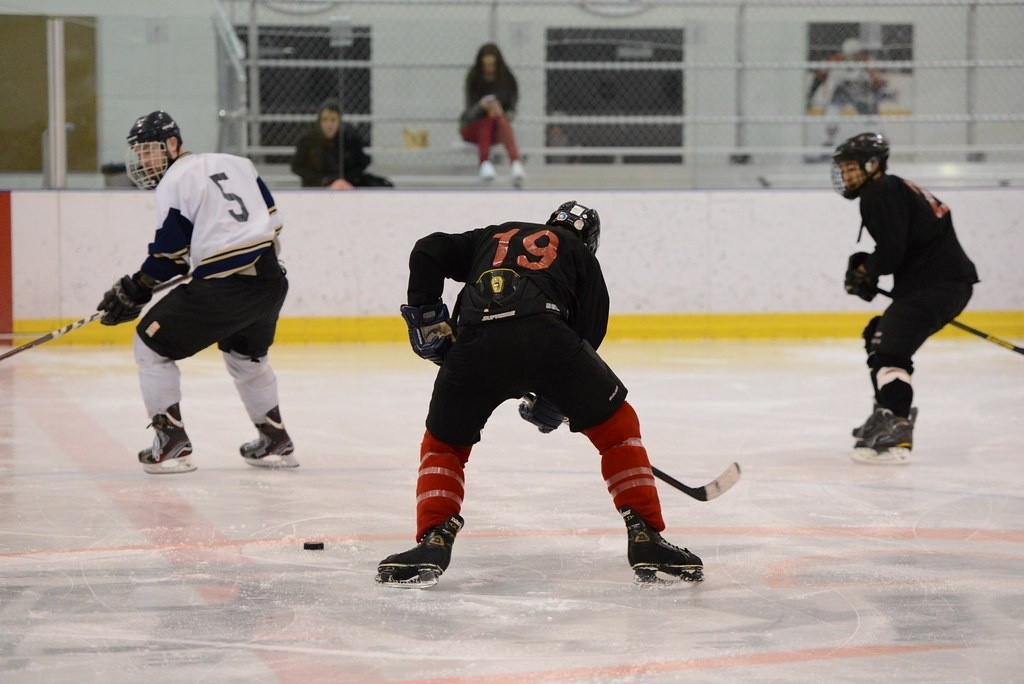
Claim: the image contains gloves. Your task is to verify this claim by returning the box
[478,94,496,110]
[519,394,565,434]
[400,297,457,366]
[846,251,879,302]
[97,275,153,324]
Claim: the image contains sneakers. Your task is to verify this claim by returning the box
[619,504,703,589]
[138,403,198,474]
[239,406,300,468]
[850,404,919,463]
[373,513,465,589]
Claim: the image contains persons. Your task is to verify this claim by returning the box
[97,110,295,463]
[376,200,703,579]
[291,98,394,190]
[459,42,525,188]
[806,39,897,164]
[831,132,981,454]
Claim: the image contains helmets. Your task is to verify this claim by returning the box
[830,132,889,200]
[546,200,601,255]
[125,110,183,190]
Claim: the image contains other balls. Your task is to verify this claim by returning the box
[304,541,326,550]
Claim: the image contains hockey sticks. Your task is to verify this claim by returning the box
[424,330,743,504]
[0,272,192,359]
[875,283,1024,358]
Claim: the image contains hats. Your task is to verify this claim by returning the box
[319,96,344,113]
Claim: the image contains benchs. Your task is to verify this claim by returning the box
[242,0,1024,184]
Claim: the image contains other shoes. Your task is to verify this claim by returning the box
[480,160,497,180]
[511,160,524,187]
